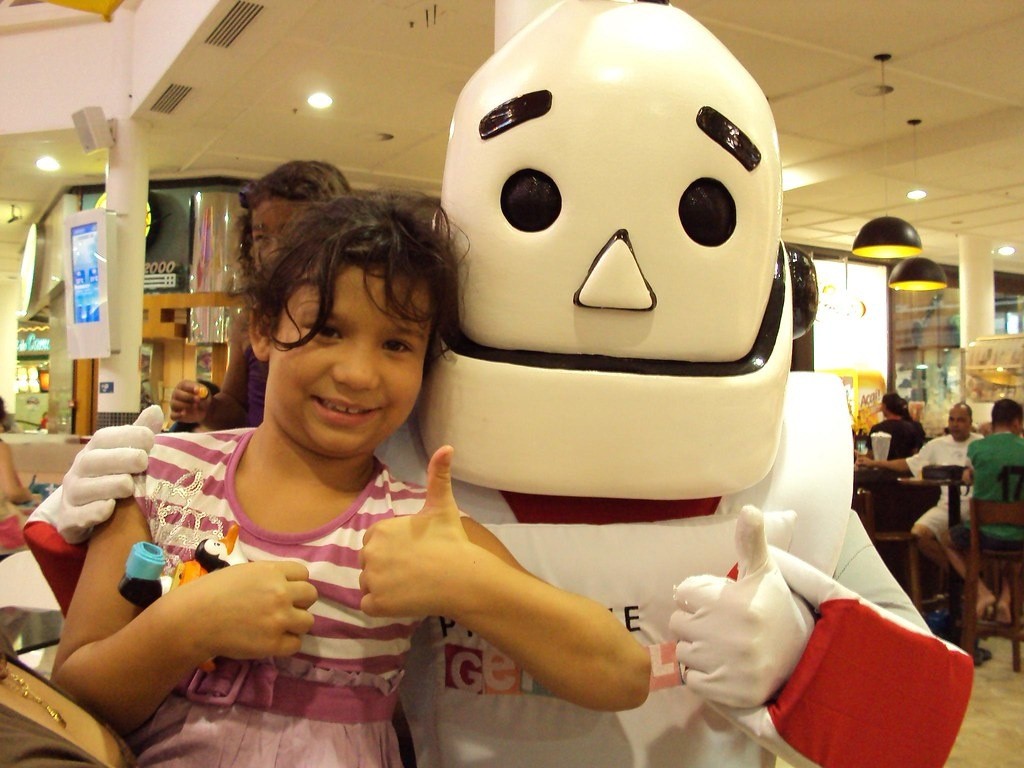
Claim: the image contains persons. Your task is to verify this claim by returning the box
[45,196,652,768]
[168,160,354,433]
[0,396,33,554]
[22,1,974,768]
[853,392,1024,623]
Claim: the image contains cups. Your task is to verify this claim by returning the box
[870,432,892,462]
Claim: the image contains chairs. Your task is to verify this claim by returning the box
[857,489,921,613]
[966,498,1024,672]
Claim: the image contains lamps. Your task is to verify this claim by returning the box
[888,119,948,290]
[71,106,116,153]
[851,53,921,259]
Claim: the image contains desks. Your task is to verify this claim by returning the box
[896,479,993,664]
